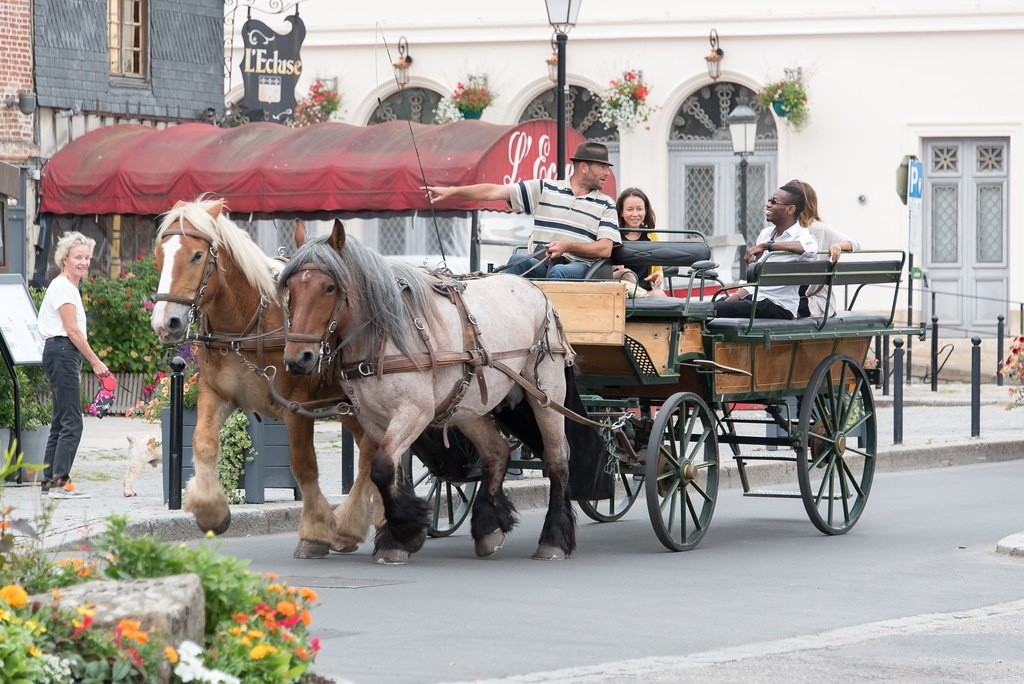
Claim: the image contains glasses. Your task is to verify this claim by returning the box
[768,199,793,205]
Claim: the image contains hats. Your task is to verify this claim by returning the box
[569,142,614,166]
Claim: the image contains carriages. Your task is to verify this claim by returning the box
[148,188,925,571]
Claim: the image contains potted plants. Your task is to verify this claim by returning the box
[161,381,302,503]
[1,353,53,482]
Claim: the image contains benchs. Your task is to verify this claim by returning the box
[609,228,905,344]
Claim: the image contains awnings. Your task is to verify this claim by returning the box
[38,119,619,276]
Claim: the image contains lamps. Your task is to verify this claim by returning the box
[545,41,560,92]
[5,91,37,115]
[704,29,724,80]
[391,37,412,85]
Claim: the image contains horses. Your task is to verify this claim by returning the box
[151,189,387,558]
[275,218,579,565]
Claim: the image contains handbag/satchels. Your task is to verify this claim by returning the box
[89,377,116,419]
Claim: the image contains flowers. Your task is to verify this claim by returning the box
[757,76,809,128]
[594,70,656,131]
[295,79,336,128]
[453,79,492,114]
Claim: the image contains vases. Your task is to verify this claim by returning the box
[464,110,481,119]
[772,100,788,117]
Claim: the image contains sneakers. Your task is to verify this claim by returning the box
[49,480,91,498]
[41,483,56,499]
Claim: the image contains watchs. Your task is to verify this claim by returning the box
[767,240,775,253]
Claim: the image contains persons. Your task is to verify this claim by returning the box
[419,143,665,295]
[35,231,109,499]
[712,180,866,318]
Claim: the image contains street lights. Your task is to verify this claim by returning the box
[727,102,759,279]
[544,1,580,178]
